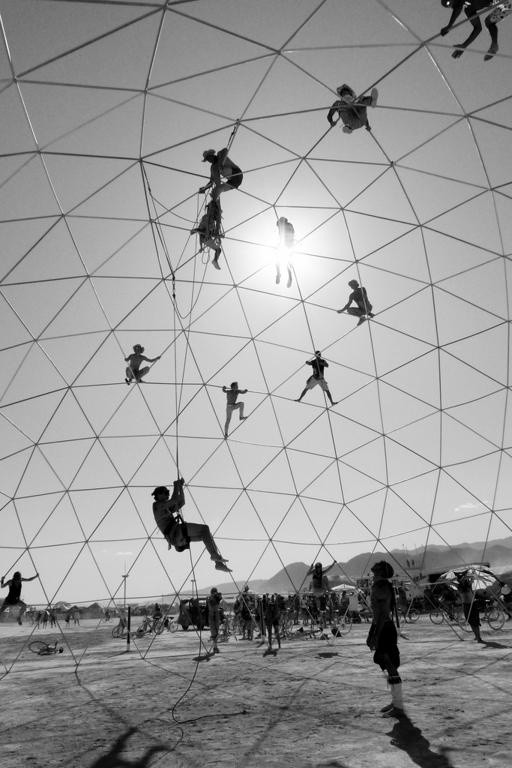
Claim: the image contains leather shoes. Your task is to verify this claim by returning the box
[210,556,229,562]
[215,564,232,572]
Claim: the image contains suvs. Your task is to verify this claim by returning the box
[179,598,224,630]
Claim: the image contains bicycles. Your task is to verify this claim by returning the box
[390,606,421,621]
[429,599,506,632]
[275,611,353,638]
[214,614,255,643]
[112,616,178,639]
[27,638,64,655]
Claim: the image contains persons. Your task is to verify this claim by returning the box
[439,0,512,61]
[26,605,81,629]
[394,569,512,643]
[276,217,295,288]
[199,148,243,198]
[337,279,374,325]
[327,84,378,134]
[0,572,39,625]
[208,561,369,654]
[222,382,248,438]
[125,344,161,384]
[365,561,404,717]
[107,603,162,638]
[191,201,225,269]
[294,350,338,405]
[151,477,233,572]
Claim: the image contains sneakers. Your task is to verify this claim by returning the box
[382,706,404,718]
[381,703,393,712]
